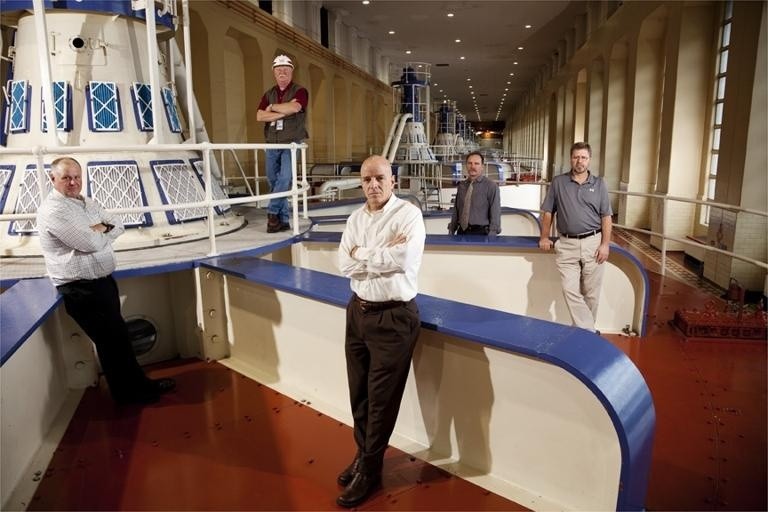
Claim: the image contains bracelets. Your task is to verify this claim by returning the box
[350,245,360,258]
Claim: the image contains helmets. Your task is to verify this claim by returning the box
[272,55,295,69]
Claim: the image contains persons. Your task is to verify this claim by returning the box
[256,54,309,233]
[337,156,426,508]
[36,157,176,403]
[448,153,501,238]
[538,142,614,335]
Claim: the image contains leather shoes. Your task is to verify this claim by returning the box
[336,471,382,507]
[337,449,362,486]
[266,213,290,233]
[150,376,176,393]
[120,393,160,404]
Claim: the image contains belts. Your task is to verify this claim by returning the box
[355,297,402,312]
[561,228,602,239]
[468,227,488,230]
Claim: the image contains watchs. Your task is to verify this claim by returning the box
[101,223,112,233]
[269,103,273,111]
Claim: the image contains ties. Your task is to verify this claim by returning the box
[460,181,473,231]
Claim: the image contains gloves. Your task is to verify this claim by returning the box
[487,231,497,242]
[449,231,455,240]
